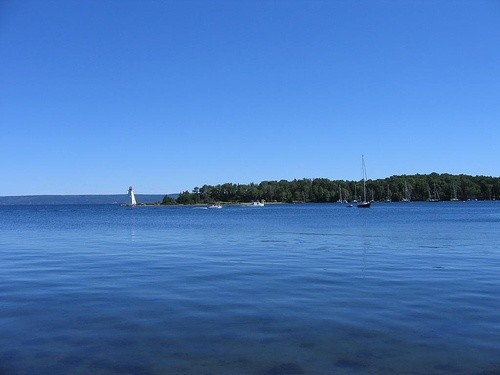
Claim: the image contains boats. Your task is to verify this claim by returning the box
[246,199,266,207]
[333,153,497,208]
[206,202,223,209]
